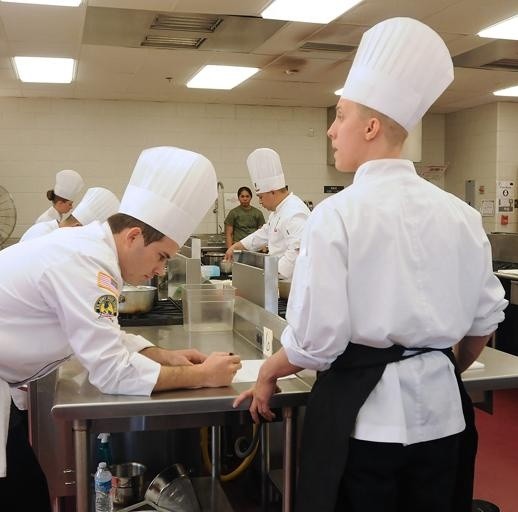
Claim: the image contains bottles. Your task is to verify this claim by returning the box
[94,462,114,512]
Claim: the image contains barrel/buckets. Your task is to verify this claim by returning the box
[93,460,146,511]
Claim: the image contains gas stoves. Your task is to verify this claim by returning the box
[117,298,184,326]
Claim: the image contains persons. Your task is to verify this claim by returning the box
[225,186,312,282]
[12,214,85,439]
[234,97,510,512]
[35,189,74,224]
[223,187,267,253]
[1,213,242,511]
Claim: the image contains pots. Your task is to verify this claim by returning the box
[203,251,229,280]
[117,285,158,315]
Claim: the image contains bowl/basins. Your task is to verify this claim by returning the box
[113,462,148,505]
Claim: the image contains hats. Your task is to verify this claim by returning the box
[71,187,121,226]
[118,146,218,248]
[54,170,83,201]
[247,148,286,193]
[341,17,454,134]
